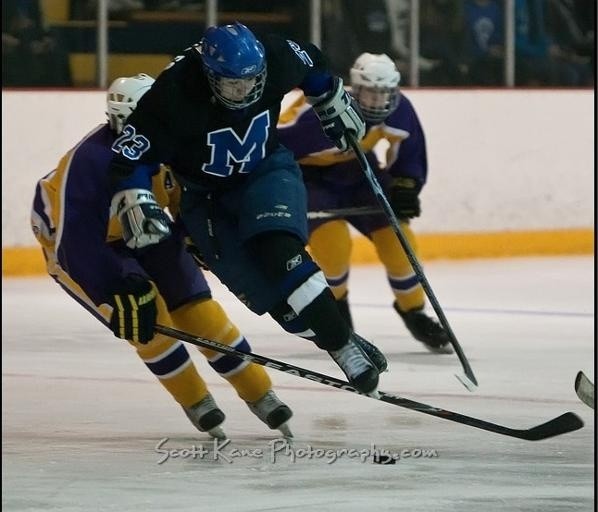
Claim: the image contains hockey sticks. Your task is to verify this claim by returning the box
[575,371,594,408]
[154,323,584,441]
[345,129,478,393]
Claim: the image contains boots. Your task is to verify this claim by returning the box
[394,300,449,347]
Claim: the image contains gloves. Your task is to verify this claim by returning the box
[105,274,157,344]
[390,185,422,220]
[121,198,173,248]
[313,83,365,152]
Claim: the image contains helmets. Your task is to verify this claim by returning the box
[201,19,268,108]
[106,72,156,134]
[350,51,402,123]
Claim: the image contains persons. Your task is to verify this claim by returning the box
[272,53,450,349]
[101,21,388,401]
[32,73,294,444]
[1,0,596,90]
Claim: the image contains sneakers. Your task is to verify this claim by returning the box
[328,331,388,392]
[245,387,293,429]
[180,389,225,432]
